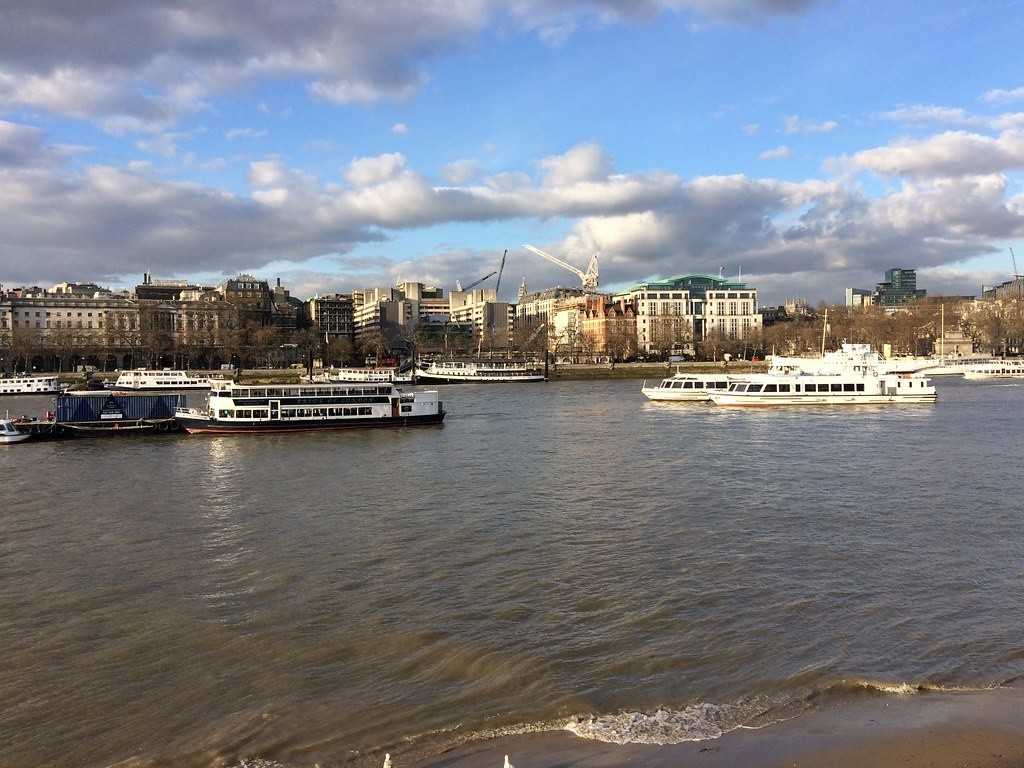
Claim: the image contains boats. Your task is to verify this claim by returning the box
[642,304,1024,420]
[298,368,412,386]
[0,371,237,446]
[175,380,448,435]
[410,359,546,387]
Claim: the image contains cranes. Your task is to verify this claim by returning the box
[453,248,509,299]
[521,242,601,288]
